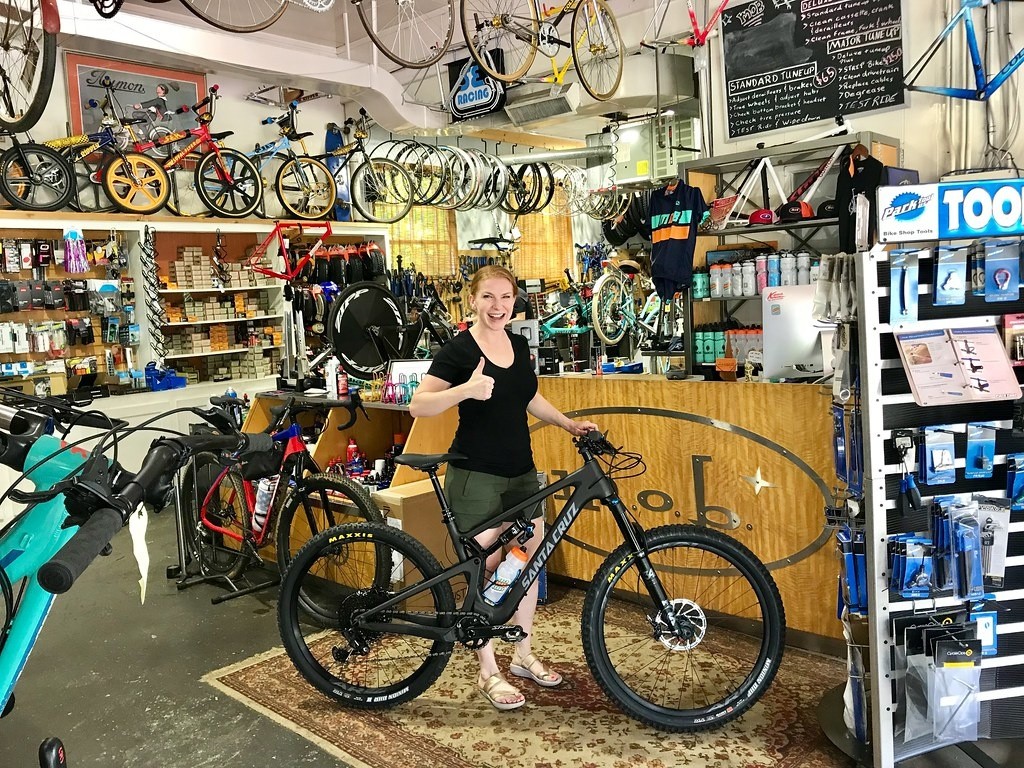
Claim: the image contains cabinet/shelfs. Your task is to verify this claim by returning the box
[219,391,461,608]
[677,130,900,382]
[0,218,396,412]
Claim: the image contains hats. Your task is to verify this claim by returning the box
[773,201,817,224]
[745,209,773,227]
[812,200,839,218]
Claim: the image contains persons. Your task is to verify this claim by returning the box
[409,264,599,709]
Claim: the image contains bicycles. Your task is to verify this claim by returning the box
[180,390,393,631]
[276,423,787,733]
[0,0,1024,382]
[0,385,274,724]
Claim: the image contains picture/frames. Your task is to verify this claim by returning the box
[62,49,213,172]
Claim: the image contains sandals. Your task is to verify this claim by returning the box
[510,652,563,686]
[476,674,525,710]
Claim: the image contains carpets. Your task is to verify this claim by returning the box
[198,574,864,768]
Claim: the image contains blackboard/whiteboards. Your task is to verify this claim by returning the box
[719,1,911,140]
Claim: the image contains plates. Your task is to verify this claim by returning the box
[303,391,330,396]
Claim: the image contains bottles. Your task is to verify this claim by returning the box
[691,249,821,298]
[695,320,763,363]
[251,474,280,540]
[225,386,251,428]
[324,433,407,496]
[336,365,349,395]
[482,545,528,604]
